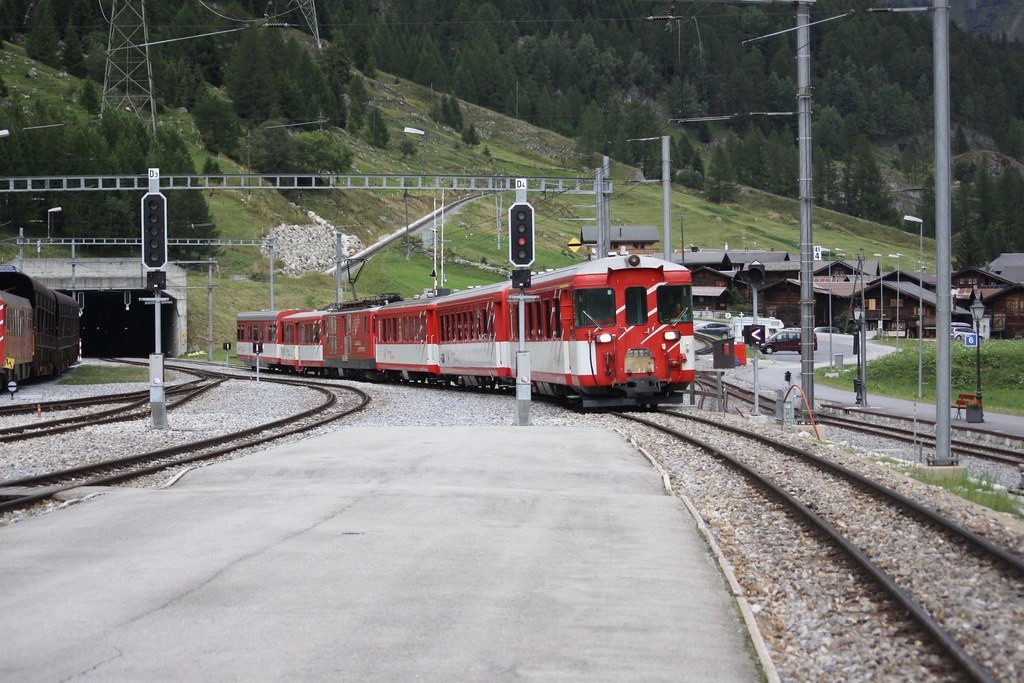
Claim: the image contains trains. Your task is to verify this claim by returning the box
[236,256,695,409]
[0,266,79,388]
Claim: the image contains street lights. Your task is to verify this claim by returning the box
[836,248,854,275]
[853,305,862,405]
[873,253,883,341]
[970,299,985,421]
[889,254,900,357]
[821,248,831,372]
[904,214,923,400]
[897,253,915,272]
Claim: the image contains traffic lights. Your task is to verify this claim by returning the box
[141,192,167,269]
[748,264,765,288]
[508,203,536,267]
[744,325,765,344]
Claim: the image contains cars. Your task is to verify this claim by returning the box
[814,327,840,334]
[950,322,985,342]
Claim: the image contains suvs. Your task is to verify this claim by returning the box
[694,322,732,339]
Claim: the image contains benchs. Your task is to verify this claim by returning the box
[951,394,976,420]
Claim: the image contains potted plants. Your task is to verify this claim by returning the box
[966,404,984,422]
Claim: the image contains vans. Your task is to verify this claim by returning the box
[758,329,818,354]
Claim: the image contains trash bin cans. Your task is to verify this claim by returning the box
[713,337,735,369]
